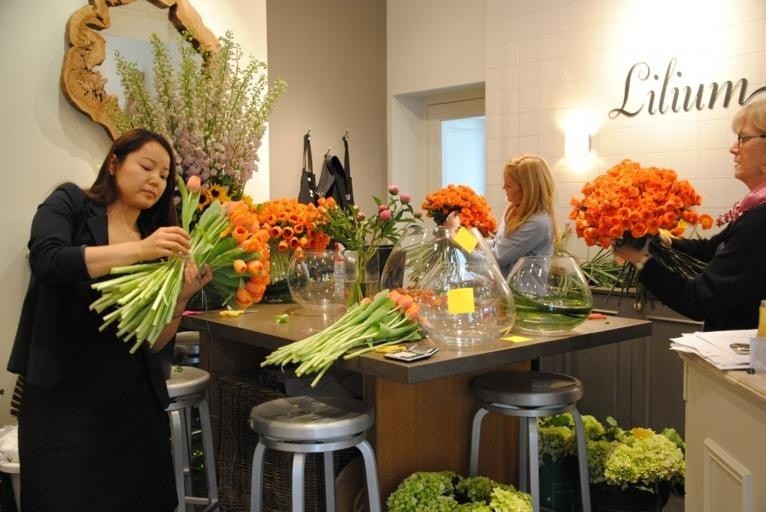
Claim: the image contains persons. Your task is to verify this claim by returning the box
[6,129,213,512]
[444,154,562,372]
[614,99,766,332]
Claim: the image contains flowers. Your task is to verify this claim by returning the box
[354,187,420,306]
[386,471,534,512]
[89,25,352,353]
[401,182,496,281]
[537,408,685,493]
[568,158,714,308]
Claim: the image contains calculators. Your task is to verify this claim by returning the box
[384,344,439,362]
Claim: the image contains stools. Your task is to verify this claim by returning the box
[249,396,381,512]
[468,370,593,511]
[164,363,220,512]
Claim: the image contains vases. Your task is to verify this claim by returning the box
[539,461,577,510]
[591,483,670,511]
[577,478,671,511]
[260,227,594,387]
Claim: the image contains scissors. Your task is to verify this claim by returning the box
[219,310,256,317]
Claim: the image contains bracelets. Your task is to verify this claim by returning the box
[636,252,653,270]
[173,312,183,318]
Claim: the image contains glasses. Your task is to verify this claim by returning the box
[737,132,765,146]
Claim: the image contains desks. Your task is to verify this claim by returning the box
[179,281,652,489]
[672,344,766,511]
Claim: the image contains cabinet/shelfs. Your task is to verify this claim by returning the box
[538,287,706,444]
